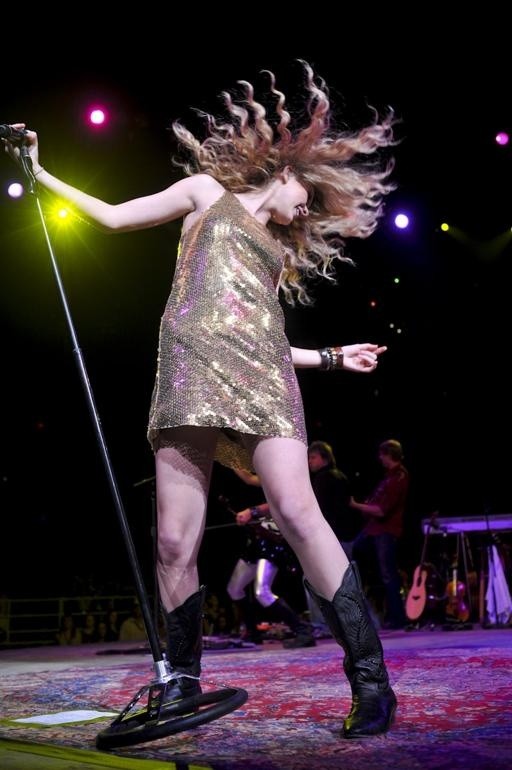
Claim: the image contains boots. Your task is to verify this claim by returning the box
[155,585,206,721]
[233,594,316,649]
[303,562,398,740]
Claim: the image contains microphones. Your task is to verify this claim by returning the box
[0,124,29,146]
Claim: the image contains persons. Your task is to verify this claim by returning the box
[304,441,381,640]
[0,58,397,741]
[57,590,227,645]
[349,439,409,629]
[225,467,316,650]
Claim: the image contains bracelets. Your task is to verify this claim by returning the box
[318,346,343,373]
[33,166,45,177]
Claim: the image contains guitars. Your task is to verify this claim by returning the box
[218,494,283,541]
[405,511,437,624]
[460,529,480,623]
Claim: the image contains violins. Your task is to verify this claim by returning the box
[445,553,471,623]
